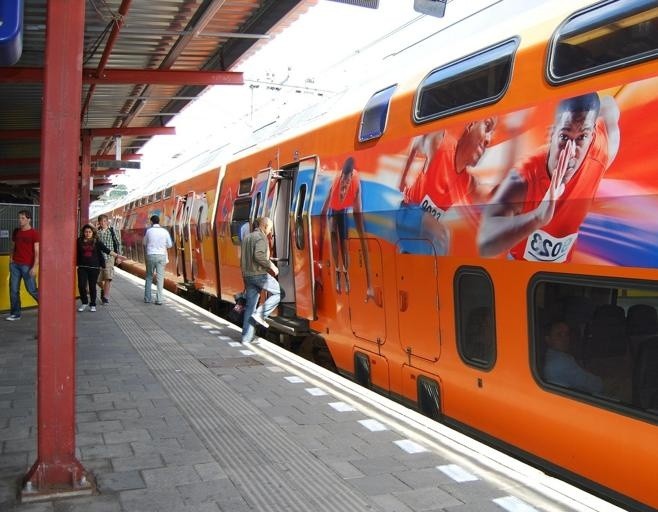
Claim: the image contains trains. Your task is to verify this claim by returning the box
[92,0,658,512]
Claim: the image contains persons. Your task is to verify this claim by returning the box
[465,306,491,361]
[97,214,123,306]
[477,92,621,263]
[6,210,39,320]
[394,115,498,257]
[77,223,127,312]
[541,320,603,395]
[319,157,374,299]
[240,216,286,344]
[142,214,173,305]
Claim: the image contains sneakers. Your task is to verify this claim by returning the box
[344,271,350,294]
[6,314,22,321]
[78,303,96,313]
[336,269,342,294]
[251,312,269,328]
[242,338,260,346]
